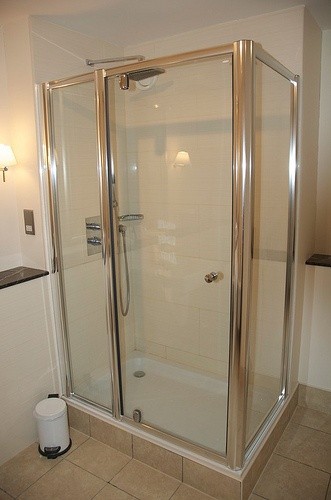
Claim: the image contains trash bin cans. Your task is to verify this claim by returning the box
[33,394,72,459]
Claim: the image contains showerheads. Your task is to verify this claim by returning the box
[126,66,165,82]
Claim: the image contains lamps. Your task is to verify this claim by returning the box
[0,144,15,182]
